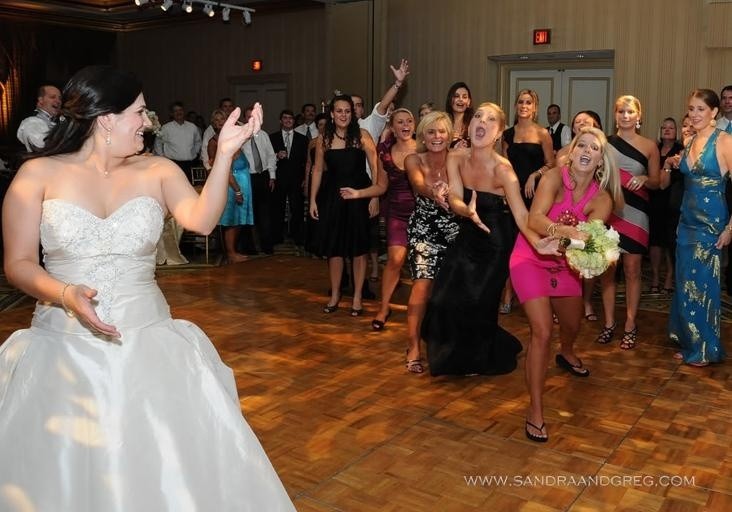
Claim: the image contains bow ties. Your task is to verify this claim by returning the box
[49,116,56,121]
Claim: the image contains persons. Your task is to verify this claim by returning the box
[0,62,298,512]
[508,126,628,443]
[1,56,732,377]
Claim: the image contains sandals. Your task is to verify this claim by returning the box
[650,283,661,292]
[662,287,674,294]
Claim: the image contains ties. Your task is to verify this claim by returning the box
[725,121,731,134]
[306,127,312,140]
[251,137,263,174]
[550,128,553,137]
[284,131,290,159]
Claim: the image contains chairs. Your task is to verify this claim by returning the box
[178,162,225,269]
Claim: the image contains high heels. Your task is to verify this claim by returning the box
[583,305,598,321]
[227,255,247,264]
[406,349,423,373]
[597,320,617,343]
[351,299,362,316]
[620,325,638,349]
[372,308,392,329]
[500,298,515,313]
[323,293,341,313]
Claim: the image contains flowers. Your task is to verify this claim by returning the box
[144,109,162,137]
[561,220,623,278]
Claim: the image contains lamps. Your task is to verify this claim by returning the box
[136,0,255,28]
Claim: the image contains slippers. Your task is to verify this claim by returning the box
[526,415,548,442]
[556,352,589,376]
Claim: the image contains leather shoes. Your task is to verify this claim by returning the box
[350,277,375,300]
[328,281,351,296]
